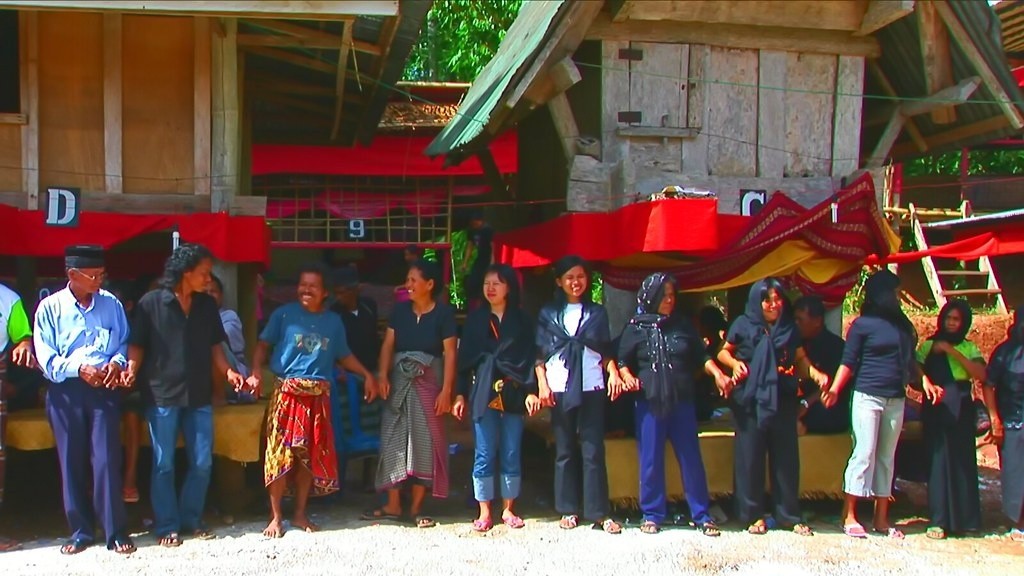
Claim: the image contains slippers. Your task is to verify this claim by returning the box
[926,525,945,539]
[360,508,399,519]
[472,517,492,530]
[1011,526,1024,541]
[872,525,904,539]
[782,521,814,536]
[499,513,524,529]
[414,515,434,528]
[748,520,767,535]
[839,521,866,536]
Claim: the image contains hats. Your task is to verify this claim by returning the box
[65,244,105,268]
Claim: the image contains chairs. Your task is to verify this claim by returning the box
[330,370,383,500]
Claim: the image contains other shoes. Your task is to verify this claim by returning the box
[0,536,22,551]
[123,487,140,502]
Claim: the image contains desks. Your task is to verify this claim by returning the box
[0,399,269,517]
[603,426,884,511]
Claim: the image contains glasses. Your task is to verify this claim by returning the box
[78,271,106,282]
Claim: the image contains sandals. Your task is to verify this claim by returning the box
[158,531,180,547]
[690,519,720,536]
[59,537,85,554]
[560,514,579,528]
[192,528,215,539]
[640,520,658,534]
[596,518,621,534]
[114,536,136,553]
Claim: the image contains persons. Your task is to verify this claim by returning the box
[0,212,1023,555]
